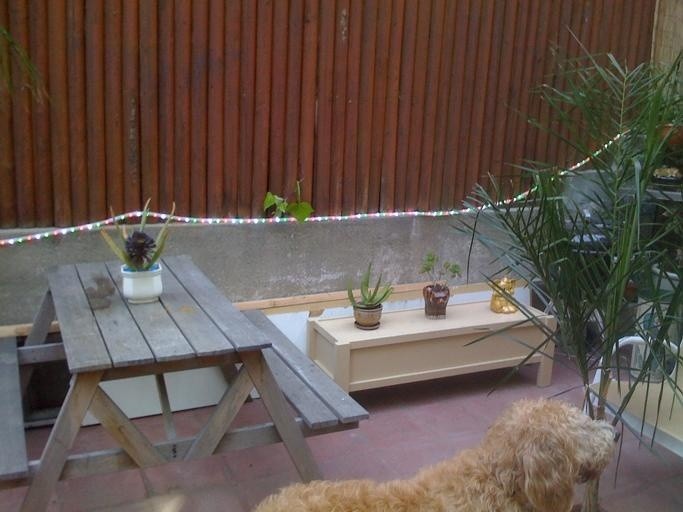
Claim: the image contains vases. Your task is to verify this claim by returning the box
[119,261,163,305]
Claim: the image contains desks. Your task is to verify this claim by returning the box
[17,250,323,512]
[307,294,560,399]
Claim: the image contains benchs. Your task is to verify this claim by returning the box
[0,334,27,489]
[225,307,369,434]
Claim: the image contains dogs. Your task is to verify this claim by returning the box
[251,394,620,512]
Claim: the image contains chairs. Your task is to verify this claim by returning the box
[581,333,681,509]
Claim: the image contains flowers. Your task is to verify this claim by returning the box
[97,197,176,270]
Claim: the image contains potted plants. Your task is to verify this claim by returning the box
[417,250,462,319]
[347,261,393,330]
